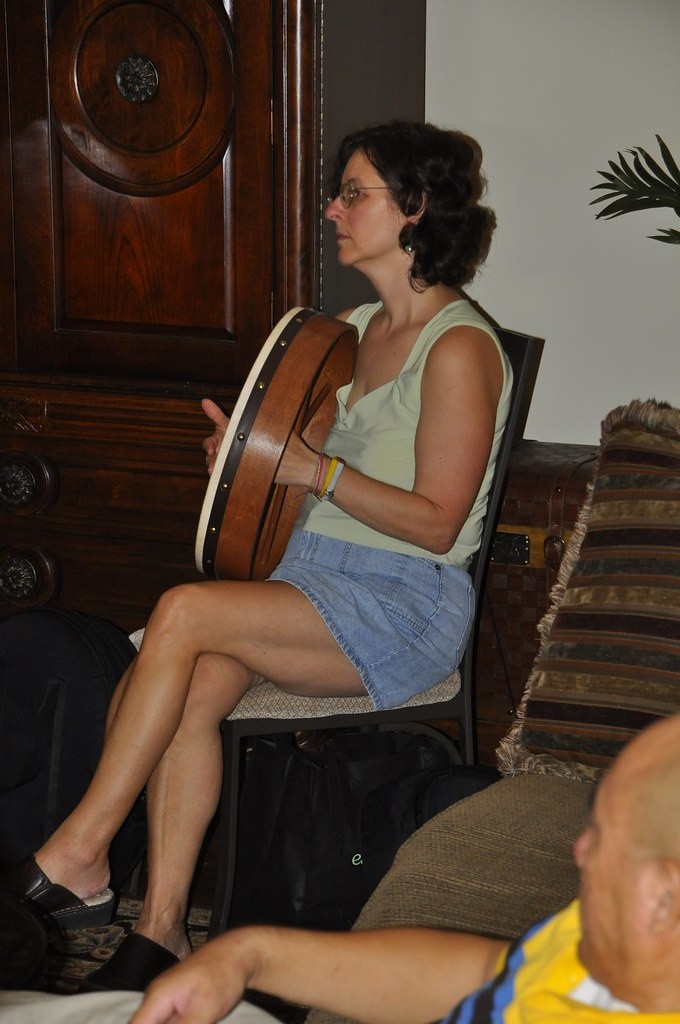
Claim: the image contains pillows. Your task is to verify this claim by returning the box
[500,404,679,781]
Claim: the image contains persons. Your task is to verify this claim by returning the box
[126,715,679,1023]
[1,124,515,990]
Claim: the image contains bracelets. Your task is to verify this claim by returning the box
[308,452,346,501]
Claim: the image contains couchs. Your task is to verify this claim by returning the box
[306,773,595,1024]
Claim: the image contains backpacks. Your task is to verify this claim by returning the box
[0,606,148,894]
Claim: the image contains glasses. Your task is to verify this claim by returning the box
[327,183,413,209]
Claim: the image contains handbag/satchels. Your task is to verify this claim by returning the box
[222,724,503,931]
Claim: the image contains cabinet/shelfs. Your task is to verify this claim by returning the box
[0,0,427,635]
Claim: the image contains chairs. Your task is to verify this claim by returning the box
[130,325,547,939]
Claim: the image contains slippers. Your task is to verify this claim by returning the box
[0,854,115,930]
[80,931,182,992]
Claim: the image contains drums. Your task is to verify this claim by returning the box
[194,305,362,581]
